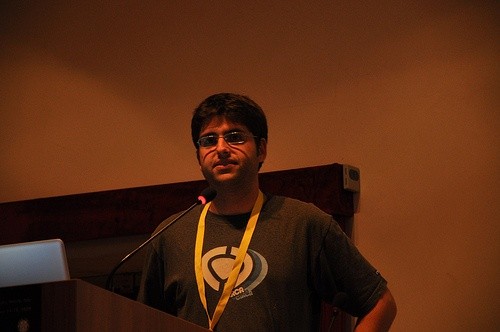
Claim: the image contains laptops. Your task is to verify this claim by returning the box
[0,239,71,288]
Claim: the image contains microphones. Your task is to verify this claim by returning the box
[103,187,218,289]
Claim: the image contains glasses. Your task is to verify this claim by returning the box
[196,132,258,148]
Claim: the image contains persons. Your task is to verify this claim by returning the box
[133,92,398,332]
